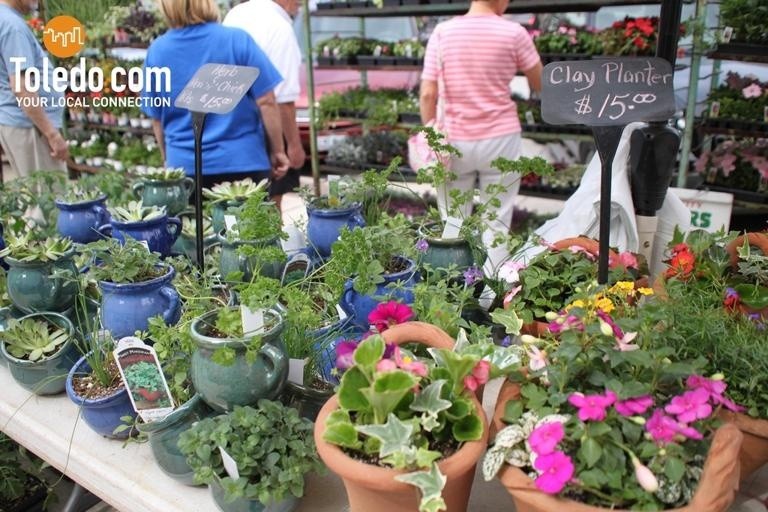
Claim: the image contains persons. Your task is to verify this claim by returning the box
[138,0,290,199]
[418,0,545,246]
[0,0,71,196]
[222,0,306,201]
